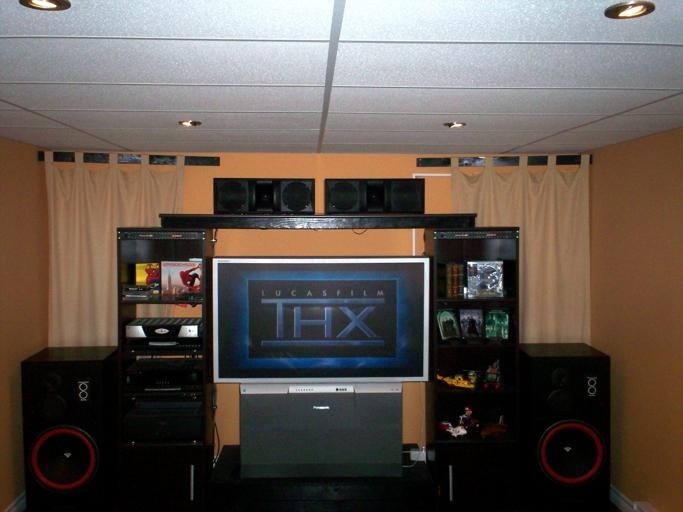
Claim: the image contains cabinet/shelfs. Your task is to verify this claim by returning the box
[423,225,520,459]
[117,225,215,452]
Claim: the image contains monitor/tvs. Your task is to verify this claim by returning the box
[210,255,432,386]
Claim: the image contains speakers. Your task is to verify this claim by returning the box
[323,177,426,215]
[518,342,611,511]
[212,176,315,216]
[20,345,126,512]
